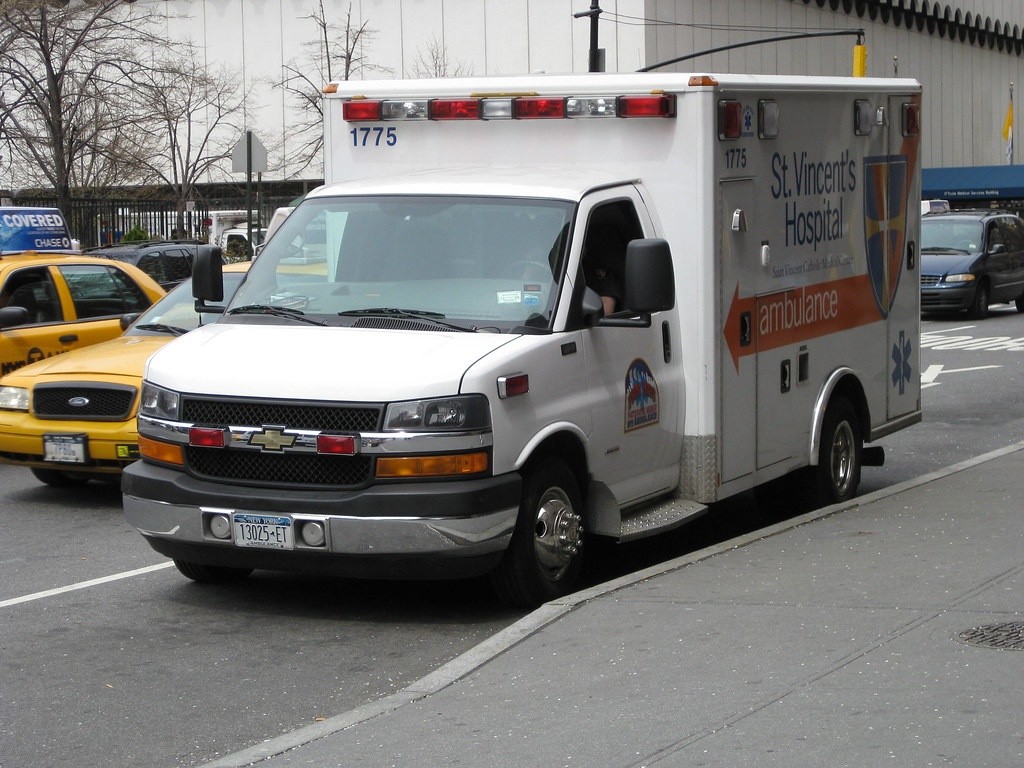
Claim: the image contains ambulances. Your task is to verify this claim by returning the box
[120,70,924,610]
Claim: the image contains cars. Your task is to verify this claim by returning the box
[921,207,1024,318]
[0,206,327,492]
[0,208,170,377]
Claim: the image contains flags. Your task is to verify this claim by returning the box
[1002,100,1014,166]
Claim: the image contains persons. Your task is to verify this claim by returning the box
[0,277,31,327]
[524,230,625,317]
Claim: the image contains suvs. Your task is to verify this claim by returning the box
[66,238,229,294]
